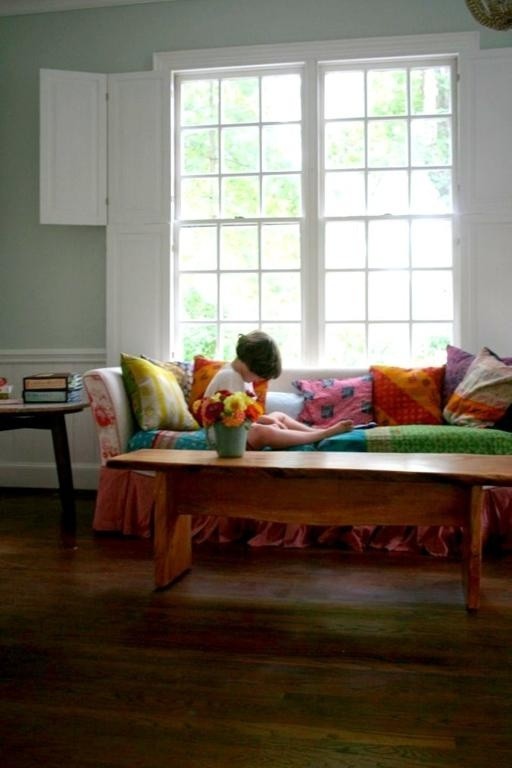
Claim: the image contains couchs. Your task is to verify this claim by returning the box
[83,366,510,552]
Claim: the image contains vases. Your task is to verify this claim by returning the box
[204,422,249,459]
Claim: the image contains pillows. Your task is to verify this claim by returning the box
[290,374,375,430]
[443,346,511,429]
[120,349,232,432]
[252,391,303,421]
[442,343,512,411]
[368,365,444,427]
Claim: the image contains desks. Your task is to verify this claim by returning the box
[0,396,89,548]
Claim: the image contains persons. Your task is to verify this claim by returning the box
[202,331,353,450]
